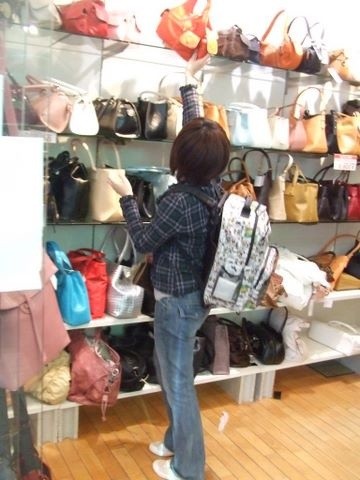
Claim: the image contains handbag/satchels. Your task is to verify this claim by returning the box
[1,0,119,41]
[268,232,360,318]
[71,140,135,224]
[115,257,157,315]
[45,240,98,322]
[308,320,360,357]
[0,68,229,151]
[67,332,123,422]
[63,247,111,319]
[216,24,251,63]
[157,0,220,66]
[226,102,271,149]
[25,350,75,404]
[216,317,267,368]
[0,251,75,390]
[248,9,360,155]
[102,326,217,394]
[106,226,146,321]
[239,305,290,366]
[45,152,92,224]
[103,165,177,220]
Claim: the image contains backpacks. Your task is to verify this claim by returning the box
[158,186,278,313]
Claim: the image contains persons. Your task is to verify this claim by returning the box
[103,50,229,480]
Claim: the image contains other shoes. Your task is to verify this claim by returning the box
[151,458,187,480]
[148,442,178,457]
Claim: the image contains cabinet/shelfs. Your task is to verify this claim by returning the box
[7,287,360,448]
[7,21,360,227]
